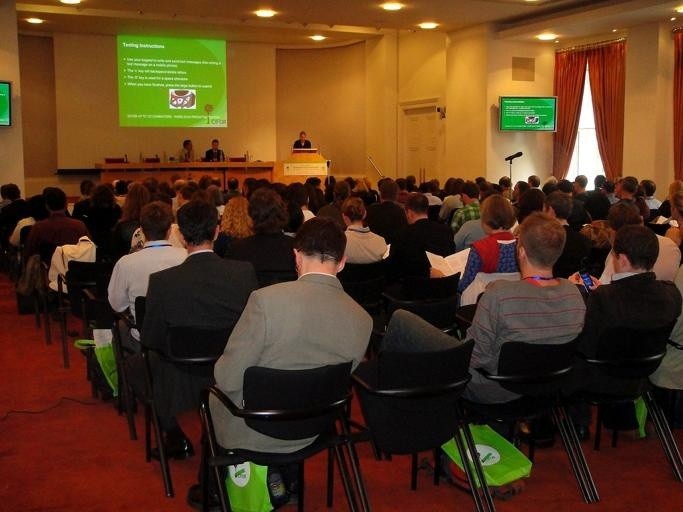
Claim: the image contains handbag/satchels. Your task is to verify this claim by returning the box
[228,463,275,511]
[75,338,118,396]
[445,458,526,498]
[441,424,533,486]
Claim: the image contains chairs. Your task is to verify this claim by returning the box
[102,156,247,162]
[0,190,682,512]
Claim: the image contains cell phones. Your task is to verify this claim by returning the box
[505,151,522,160]
[578,267,593,293]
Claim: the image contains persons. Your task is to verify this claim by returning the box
[0,176,682,510]
[177,139,194,161]
[205,139,224,162]
[293,131,310,148]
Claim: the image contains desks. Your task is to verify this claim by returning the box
[94,161,276,194]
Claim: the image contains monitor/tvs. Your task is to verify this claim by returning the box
[0,81,12,127]
[498,96,558,132]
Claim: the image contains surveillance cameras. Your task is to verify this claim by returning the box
[437,106,446,119]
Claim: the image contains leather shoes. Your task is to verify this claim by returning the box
[152,439,194,460]
[562,418,591,442]
[281,474,300,505]
[187,483,223,512]
